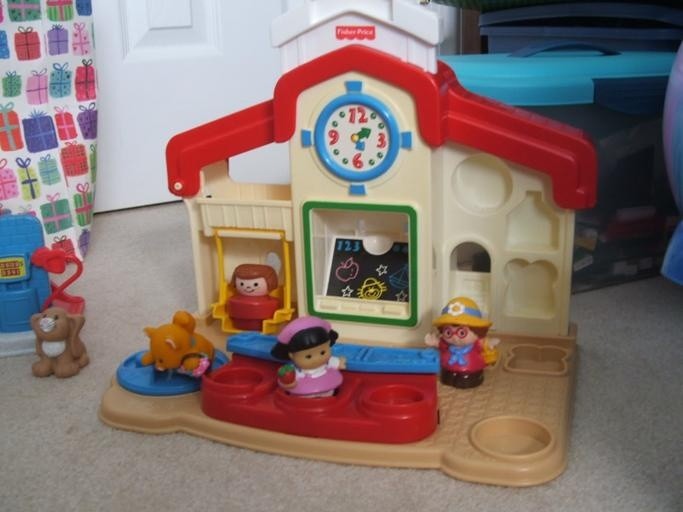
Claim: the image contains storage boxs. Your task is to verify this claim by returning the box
[475,1,682,54]
[432,44,679,301]
